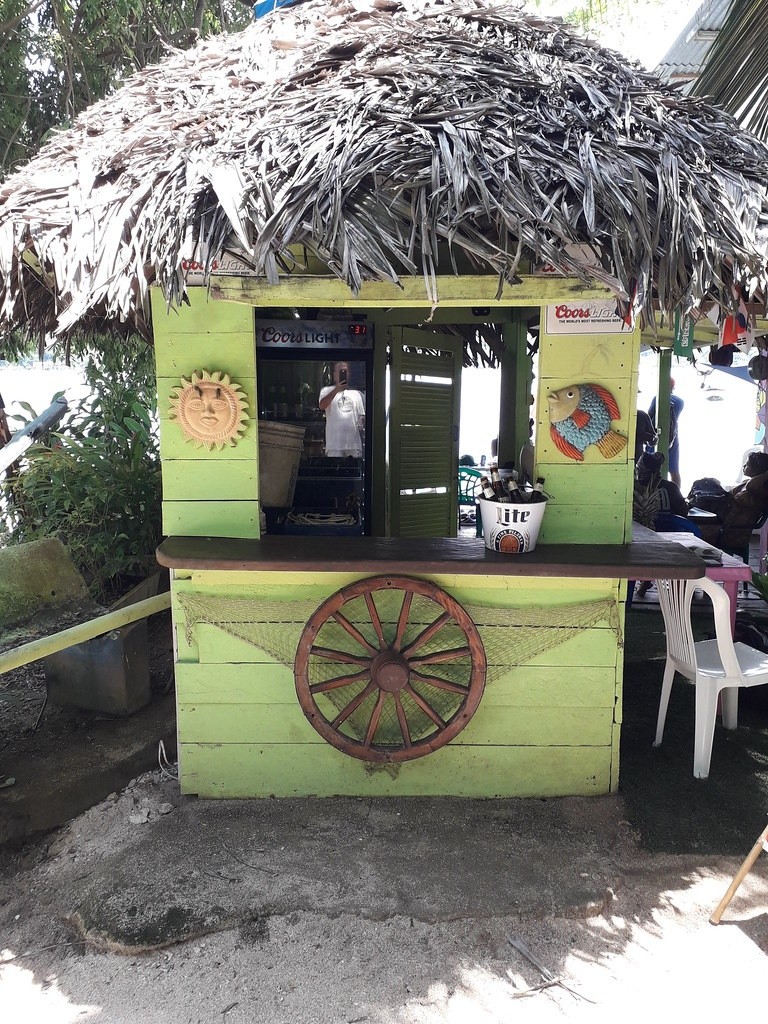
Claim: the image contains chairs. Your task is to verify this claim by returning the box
[627,515,702,606]
[652,576,768,779]
[718,511,768,592]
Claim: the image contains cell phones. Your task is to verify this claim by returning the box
[339,370,347,385]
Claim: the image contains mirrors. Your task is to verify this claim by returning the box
[257,345,369,534]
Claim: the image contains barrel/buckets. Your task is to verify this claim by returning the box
[477,490,547,553]
[258,420,307,507]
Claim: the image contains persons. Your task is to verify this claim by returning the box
[686,452,768,523]
[635,377,689,517]
[319,362,366,456]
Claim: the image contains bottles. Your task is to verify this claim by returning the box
[481,476,488,481]
[279,386,288,418]
[295,385,304,418]
[481,480,499,503]
[526,477,545,504]
[269,385,278,417]
[490,467,510,503]
[481,454,486,465]
[506,476,526,504]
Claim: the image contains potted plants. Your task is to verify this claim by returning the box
[0,389,163,613]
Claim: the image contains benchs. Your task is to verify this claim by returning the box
[0,536,151,717]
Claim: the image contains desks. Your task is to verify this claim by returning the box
[627,532,753,715]
